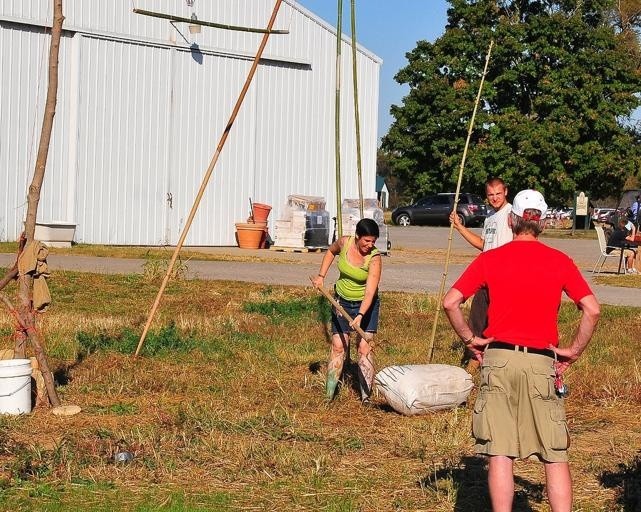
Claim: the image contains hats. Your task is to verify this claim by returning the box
[512,190,547,220]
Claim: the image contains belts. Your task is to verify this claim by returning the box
[489,340,555,358]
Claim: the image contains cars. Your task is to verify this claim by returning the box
[544,206,619,224]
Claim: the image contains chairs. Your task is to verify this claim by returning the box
[592,225,628,277]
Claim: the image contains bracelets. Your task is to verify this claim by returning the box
[464,334,476,346]
[318,274,326,279]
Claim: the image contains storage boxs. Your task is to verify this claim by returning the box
[34,225,76,242]
[335,198,388,253]
[273,194,330,248]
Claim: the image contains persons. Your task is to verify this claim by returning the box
[449,178,512,367]
[310,218,384,410]
[442,189,601,512]
[588,195,641,275]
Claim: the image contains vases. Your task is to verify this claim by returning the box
[235,203,273,250]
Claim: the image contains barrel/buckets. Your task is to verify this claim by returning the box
[0,359,35,415]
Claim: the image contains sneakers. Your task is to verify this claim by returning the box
[627,268,641,275]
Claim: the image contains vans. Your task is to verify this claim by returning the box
[388,189,488,228]
